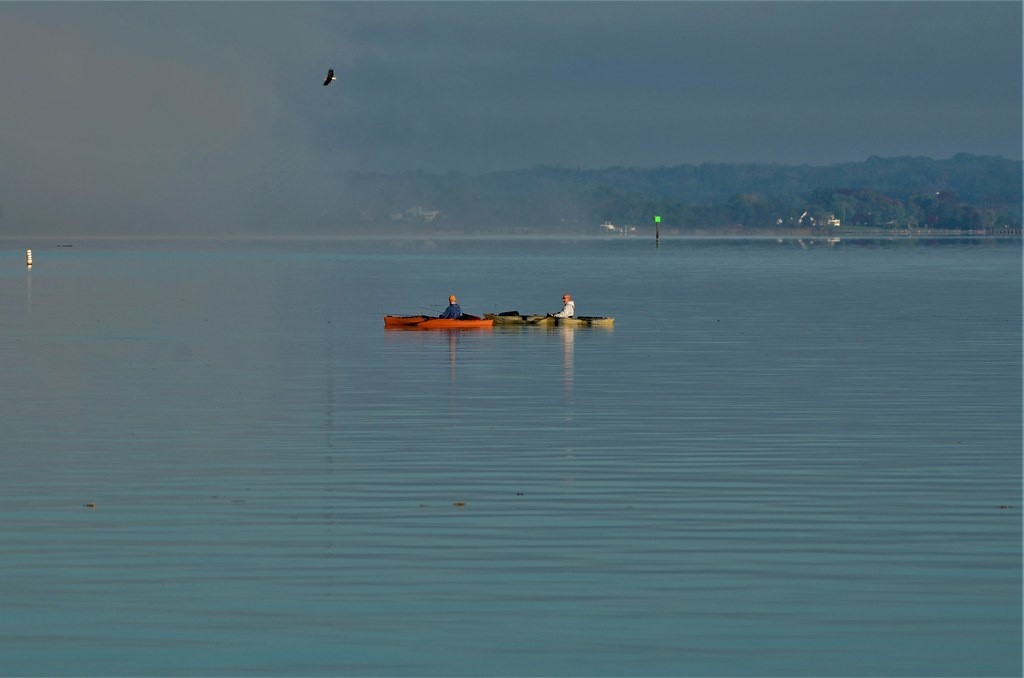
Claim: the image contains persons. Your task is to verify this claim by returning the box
[547,294,574,318]
[439,295,461,320]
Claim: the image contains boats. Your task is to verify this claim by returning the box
[483,312,616,326]
[383,315,494,329]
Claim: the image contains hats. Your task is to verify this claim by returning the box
[449,295,456,301]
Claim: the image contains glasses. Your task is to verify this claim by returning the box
[562,298,566,299]
